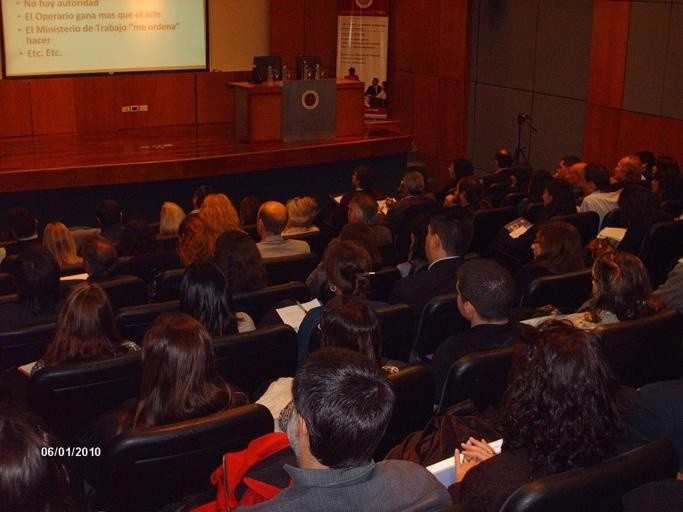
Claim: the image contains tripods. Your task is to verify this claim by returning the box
[512,129,525,164]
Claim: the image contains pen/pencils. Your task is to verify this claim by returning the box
[294,299,309,316]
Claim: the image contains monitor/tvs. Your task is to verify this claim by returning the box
[253,55,281,81]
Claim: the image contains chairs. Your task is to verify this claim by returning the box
[254,56,282,84]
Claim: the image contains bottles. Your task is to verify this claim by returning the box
[281,63,288,83]
[266,65,274,86]
[314,63,321,80]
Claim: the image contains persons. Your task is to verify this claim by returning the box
[362,81,387,109]
[343,67,358,82]
[363,78,381,97]
[2,146,683,511]
